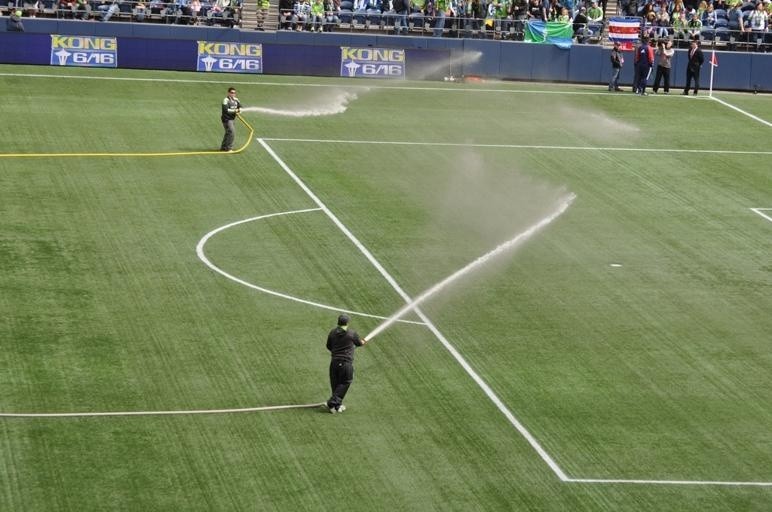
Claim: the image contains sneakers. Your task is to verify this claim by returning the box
[324,402,346,413]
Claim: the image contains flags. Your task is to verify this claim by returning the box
[523,19,574,49]
[608,16,641,45]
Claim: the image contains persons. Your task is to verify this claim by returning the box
[654,40,675,93]
[278,1,607,45]
[608,41,624,93]
[1,1,243,33]
[325,314,366,413]
[614,1,771,53]
[632,38,651,92]
[219,87,242,152]
[255,1,270,31]
[636,36,654,95]
[684,41,704,94]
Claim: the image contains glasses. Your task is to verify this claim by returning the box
[230,91,235,94]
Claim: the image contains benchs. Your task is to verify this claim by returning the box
[23,0,772,51]
